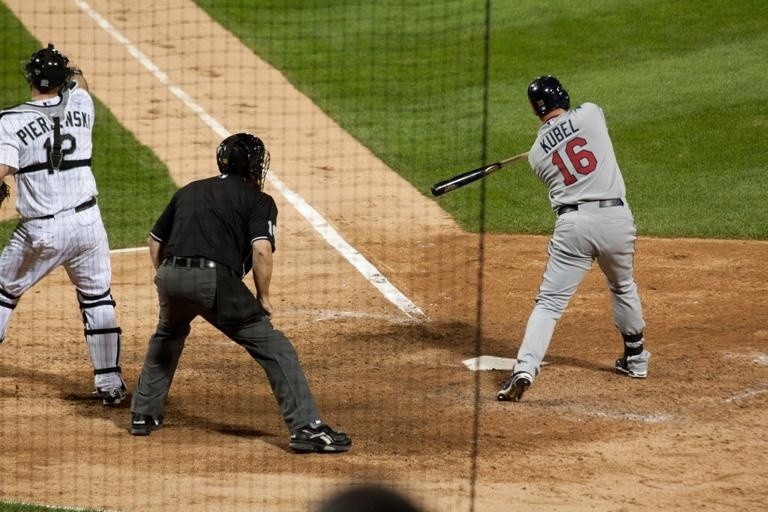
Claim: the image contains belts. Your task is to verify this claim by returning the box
[552,196,623,214]
[24,196,98,220]
[165,255,216,269]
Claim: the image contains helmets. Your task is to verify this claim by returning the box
[527,74,572,120]
[216,132,270,192]
[25,46,70,92]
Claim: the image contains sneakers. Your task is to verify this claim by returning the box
[615,350,651,379]
[130,412,164,437]
[289,420,352,453]
[97,386,124,404]
[497,371,535,401]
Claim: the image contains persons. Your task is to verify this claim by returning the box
[0,43,127,407]
[316,487,417,512]
[127,132,351,453]
[496,75,651,403]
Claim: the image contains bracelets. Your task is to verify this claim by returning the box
[70,70,81,75]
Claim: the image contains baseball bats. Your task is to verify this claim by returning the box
[431,151,530,196]
